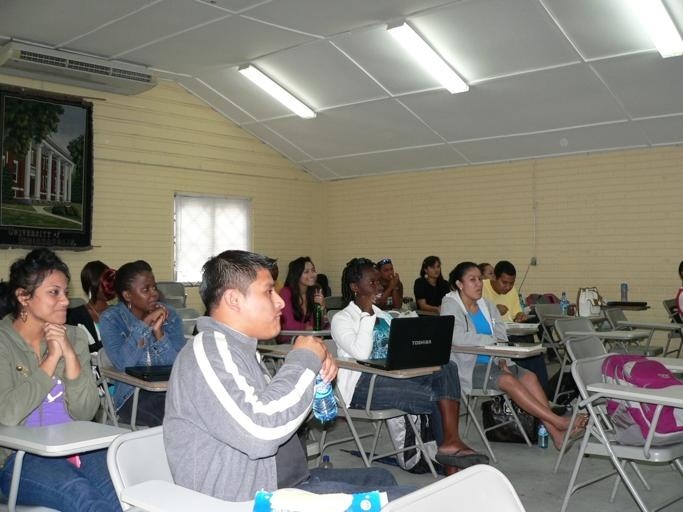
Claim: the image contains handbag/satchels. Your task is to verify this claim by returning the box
[481,400,539,445]
[526,293,574,316]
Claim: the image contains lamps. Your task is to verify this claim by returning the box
[386,22,469,96]
[238,64,316,122]
[627,0,683,60]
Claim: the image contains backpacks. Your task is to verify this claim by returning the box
[384,413,445,474]
[548,368,580,416]
[579,289,602,316]
[602,355,683,446]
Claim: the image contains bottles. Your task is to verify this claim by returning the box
[313,303,322,331]
[560,292,569,315]
[312,371,339,425]
[621,283,628,302]
[537,424,549,448]
[319,455,334,472]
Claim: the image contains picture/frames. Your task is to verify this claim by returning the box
[0,84,92,247]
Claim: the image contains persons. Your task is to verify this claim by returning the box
[440,262,588,452]
[330,258,489,476]
[99,261,186,428]
[413,256,528,325]
[265,257,332,343]
[69,261,111,394]
[0,247,120,511]
[675,261,683,322]
[163,249,421,500]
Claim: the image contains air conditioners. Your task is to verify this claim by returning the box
[0,41,158,96]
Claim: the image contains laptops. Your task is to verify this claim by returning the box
[355,314,455,373]
[125,365,172,383]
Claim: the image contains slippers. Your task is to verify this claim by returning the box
[561,414,588,453]
[435,448,489,468]
